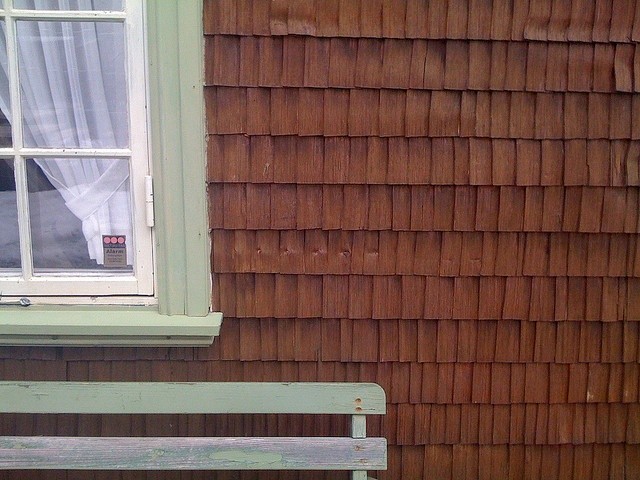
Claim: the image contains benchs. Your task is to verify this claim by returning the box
[0,381,388,480]
